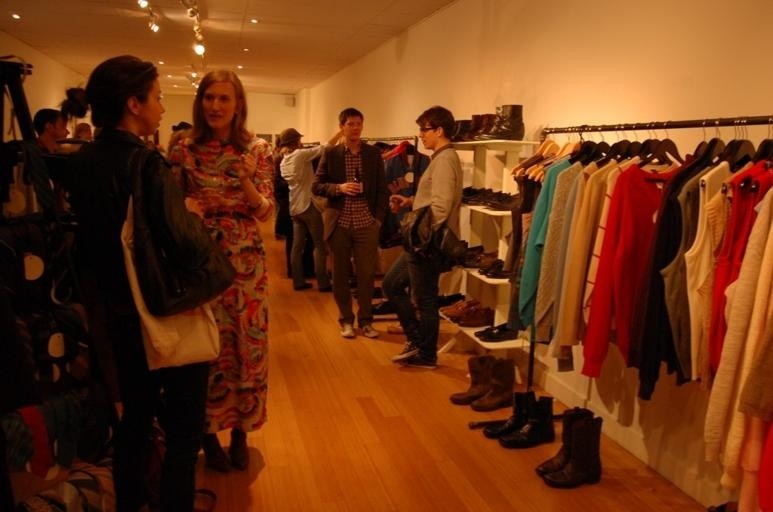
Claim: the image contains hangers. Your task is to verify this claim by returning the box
[510,116,773,186]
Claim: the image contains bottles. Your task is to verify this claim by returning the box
[353,168,365,197]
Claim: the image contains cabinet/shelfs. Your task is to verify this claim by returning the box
[438,140,535,387]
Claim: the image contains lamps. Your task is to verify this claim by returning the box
[138,0,149,8]
[179,0,205,55]
[147,7,161,33]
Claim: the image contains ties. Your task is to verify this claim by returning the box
[96,456,113,471]
[0,301,94,412]
[37,381,112,465]
[65,468,105,512]
[0,215,50,320]
[18,496,53,512]
[71,458,116,512]
[37,481,84,512]
[0,410,35,472]
[16,404,56,480]
[8,139,56,211]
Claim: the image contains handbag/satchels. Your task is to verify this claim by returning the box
[120,194,221,372]
[123,145,239,319]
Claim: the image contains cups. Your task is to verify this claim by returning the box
[390,197,402,216]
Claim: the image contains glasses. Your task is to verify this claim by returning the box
[419,127,436,132]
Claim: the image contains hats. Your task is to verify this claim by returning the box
[279,127,304,146]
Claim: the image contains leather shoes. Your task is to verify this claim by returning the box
[478,258,504,275]
[474,323,510,337]
[480,324,519,343]
[459,239,499,268]
[438,293,497,326]
[460,186,521,211]
[485,261,511,279]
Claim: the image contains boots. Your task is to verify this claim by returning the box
[471,113,497,140]
[499,396,555,449]
[482,106,503,139]
[483,390,538,439]
[449,355,497,405]
[543,415,604,489]
[462,114,482,141]
[480,104,525,140]
[449,119,472,141]
[535,405,595,477]
[470,357,516,412]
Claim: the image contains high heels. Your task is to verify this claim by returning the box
[228,430,250,470]
[200,432,232,473]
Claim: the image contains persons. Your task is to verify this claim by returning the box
[25,107,83,215]
[60,51,210,512]
[381,104,464,370]
[70,122,94,152]
[170,117,198,155]
[168,68,277,472]
[311,107,391,338]
[273,135,316,282]
[278,127,345,292]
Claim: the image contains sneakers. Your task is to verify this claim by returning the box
[391,340,421,364]
[294,282,313,291]
[398,356,438,370]
[338,322,356,338]
[357,324,380,338]
[319,285,334,292]
